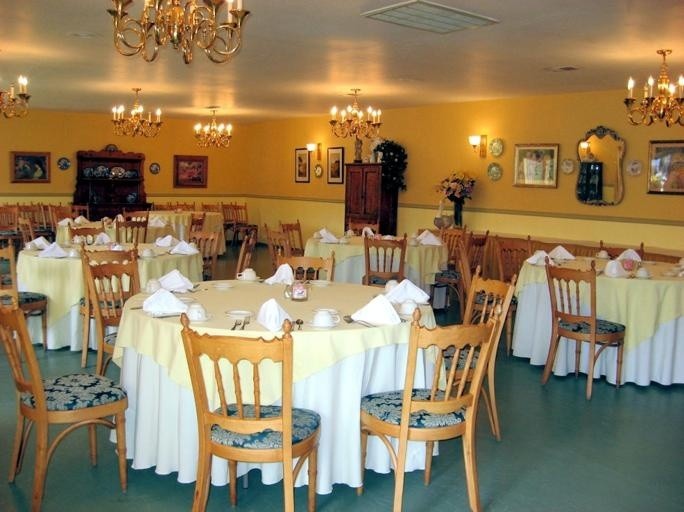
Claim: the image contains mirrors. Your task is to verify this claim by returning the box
[575,124,628,206]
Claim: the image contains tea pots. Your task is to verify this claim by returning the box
[82,167,93,177]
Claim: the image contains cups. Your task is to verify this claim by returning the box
[186,303,209,320]
[291,282,308,302]
[312,230,381,244]
[145,279,160,294]
[384,280,398,292]
[398,299,417,315]
[236,268,256,280]
[595,250,649,278]
[313,310,340,327]
[651,176,659,189]
[24,234,157,258]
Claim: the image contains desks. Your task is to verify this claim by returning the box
[16,216,203,355]
[122,277,433,491]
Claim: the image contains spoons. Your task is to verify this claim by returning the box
[295,318,303,330]
[343,315,374,327]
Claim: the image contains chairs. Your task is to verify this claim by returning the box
[0,199,89,354]
[1,296,129,511]
[494,234,683,401]
[304,232,447,286]
[235,233,256,277]
[277,247,335,281]
[182,226,220,280]
[122,200,257,256]
[81,241,140,378]
[263,219,317,279]
[442,265,516,443]
[181,311,321,511]
[430,226,492,320]
[357,303,502,512]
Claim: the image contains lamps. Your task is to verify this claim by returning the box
[580,140,597,159]
[101,0,251,67]
[191,109,232,150]
[621,47,683,131]
[0,74,31,121]
[110,88,165,138]
[466,131,487,160]
[327,86,383,142]
[306,142,322,160]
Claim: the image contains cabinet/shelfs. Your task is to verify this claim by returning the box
[577,161,604,201]
[74,144,152,222]
[342,160,400,238]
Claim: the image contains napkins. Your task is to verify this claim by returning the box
[383,278,430,304]
[350,294,401,326]
[263,263,295,287]
[258,299,293,333]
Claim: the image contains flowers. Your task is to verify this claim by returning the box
[432,168,476,226]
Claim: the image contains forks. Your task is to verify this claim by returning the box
[230,319,249,330]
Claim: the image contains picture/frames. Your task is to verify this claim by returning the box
[512,143,560,188]
[294,147,310,183]
[646,138,684,196]
[8,149,52,184]
[172,154,209,189]
[327,146,345,184]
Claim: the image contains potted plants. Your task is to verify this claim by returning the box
[370,139,409,193]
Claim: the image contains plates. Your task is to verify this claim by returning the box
[210,283,234,288]
[486,162,502,181]
[489,137,502,157]
[625,160,642,176]
[307,280,329,286]
[178,296,197,303]
[224,310,253,317]
[313,308,337,314]
[560,159,575,175]
[149,163,159,174]
[93,163,108,178]
[57,157,70,170]
[659,271,679,276]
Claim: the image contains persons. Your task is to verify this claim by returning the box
[178,164,202,182]
[519,151,553,180]
[15,158,47,179]
